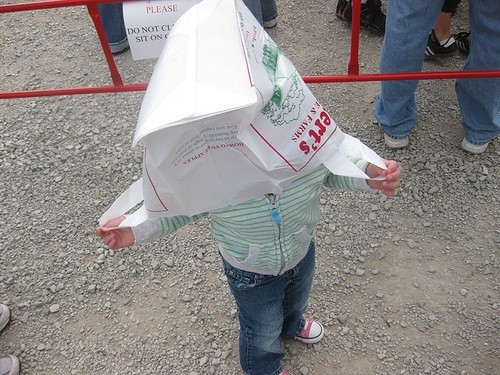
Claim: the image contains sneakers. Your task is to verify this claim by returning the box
[293,315,326,345]
[422,28,470,62]
[344,0,388,34]
[278,369,293,375]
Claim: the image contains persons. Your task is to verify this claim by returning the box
[97,0,277,56]
[336,0,500,154]
[96,133,401,375]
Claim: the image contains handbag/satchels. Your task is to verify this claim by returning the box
[98,0,390,233]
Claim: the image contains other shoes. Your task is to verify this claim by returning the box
[108,35,130,55]
[376,118,409,149]
[461,135,493,155]
[336,0,348,18]
[0,355,22,375]
[262,16,278,28]
[0,304,11,333]
